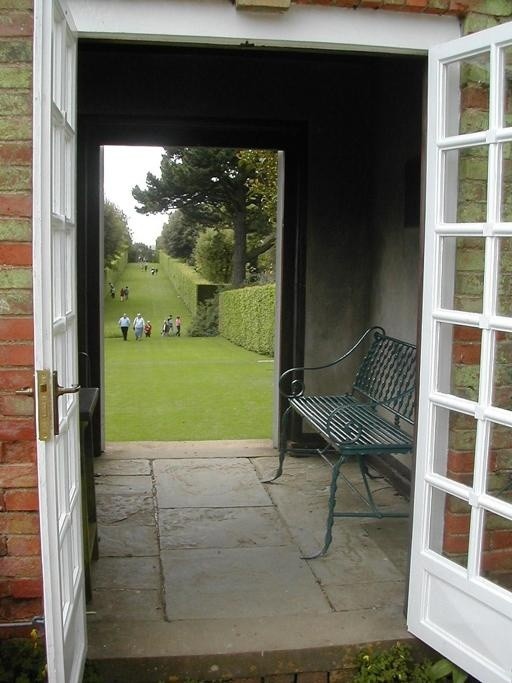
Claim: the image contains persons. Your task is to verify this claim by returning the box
[132,313,146,342]
[118,313,131,341]
[175,315,181,336]
[119,287,124,302]
[144,320,152,338]
[160,314,174,336]
[137,255,159,276]
[123,286,130,303]
[111,286,115,298]
[109,282,114,296]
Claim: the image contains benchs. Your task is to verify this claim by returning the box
[261,326,416,559]
